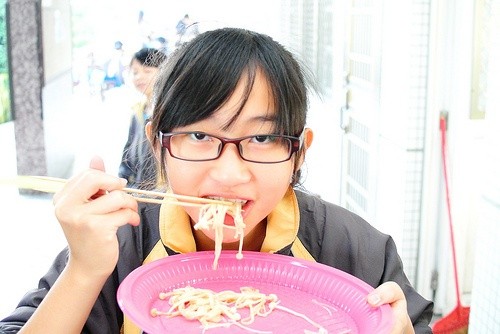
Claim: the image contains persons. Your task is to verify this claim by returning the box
[117,48,167,191]
[0,26,437,334]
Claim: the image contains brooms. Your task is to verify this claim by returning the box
[431,110,470,334]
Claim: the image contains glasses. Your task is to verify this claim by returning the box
[152,122,302,164]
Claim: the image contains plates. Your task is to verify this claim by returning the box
[116,252,394,334]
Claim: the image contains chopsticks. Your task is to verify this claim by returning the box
[18,175,234,211]
